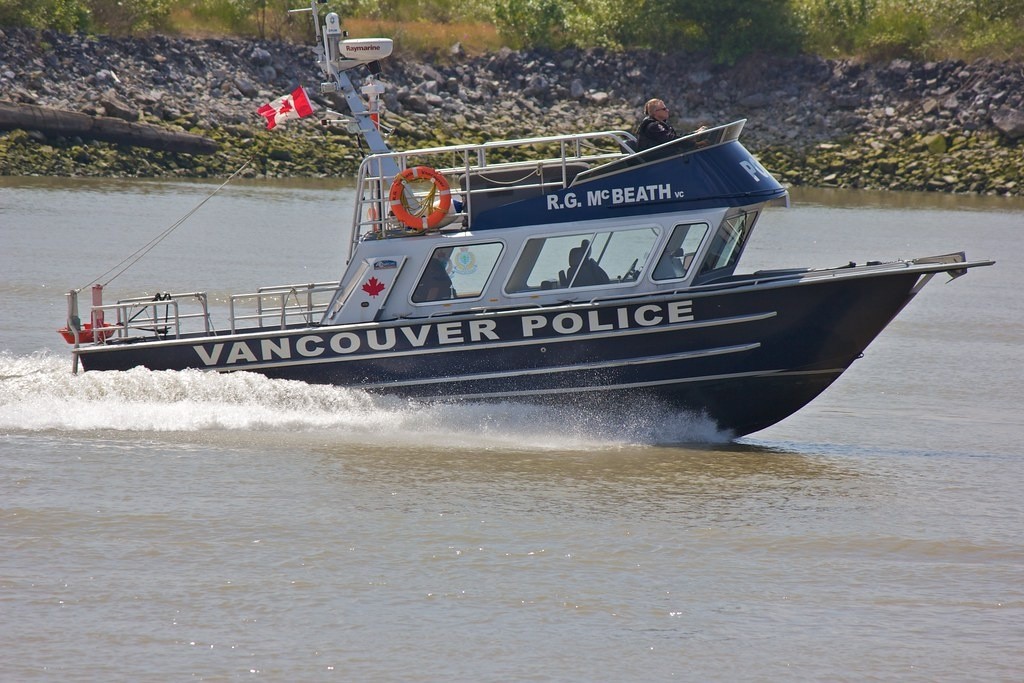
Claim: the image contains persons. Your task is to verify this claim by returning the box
[567,239,611,291]
[636,98,711,155]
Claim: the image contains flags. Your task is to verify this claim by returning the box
[257,85,315,130]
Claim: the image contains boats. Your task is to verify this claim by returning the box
[53,1,1001,446]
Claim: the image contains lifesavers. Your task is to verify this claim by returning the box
[367,207,380,232]
[388,166,452,230]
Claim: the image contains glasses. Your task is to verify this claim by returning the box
[656,107,667,111]
